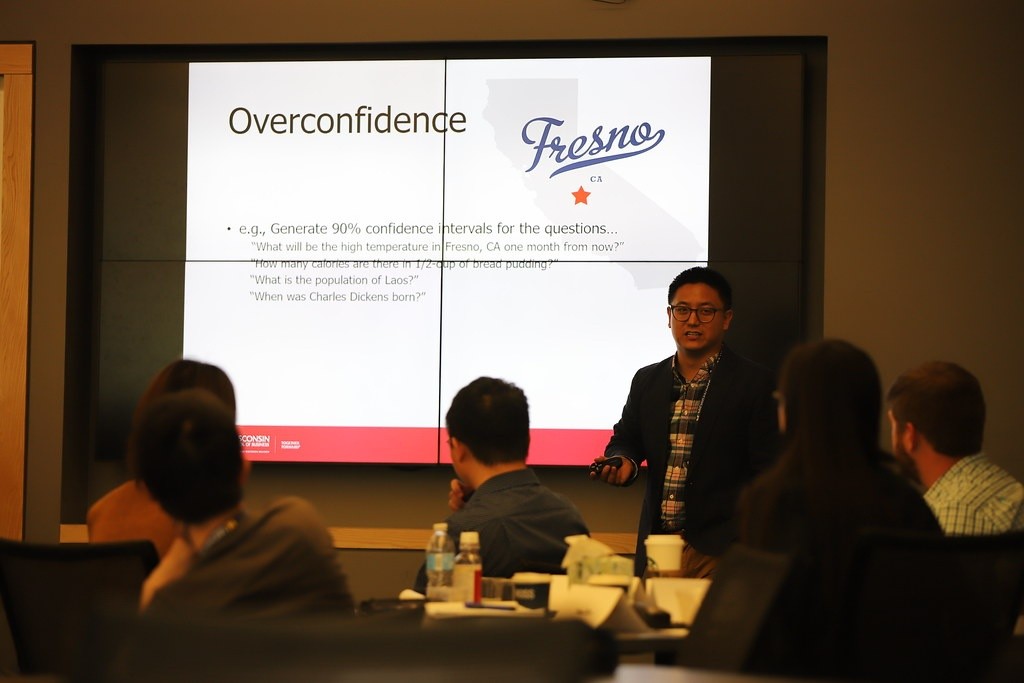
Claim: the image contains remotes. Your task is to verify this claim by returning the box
[589,457,622,473]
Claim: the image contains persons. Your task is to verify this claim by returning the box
[669,337,957,683]
[134,388,359,626]
[885,359,1024,648]
[86,361,237,571]
[589,266,772,578]
[412,375,594,602]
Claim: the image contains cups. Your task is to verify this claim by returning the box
[512,571,551,616]
[643,533,686,580]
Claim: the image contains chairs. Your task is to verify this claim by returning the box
[813,528,1024,681]
[677,544,793,673]
[0,537,160,676]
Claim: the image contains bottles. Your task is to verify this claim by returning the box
[427,523,457,601]
[452,531,485,606]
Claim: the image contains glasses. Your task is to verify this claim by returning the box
[668,303,728,324]
